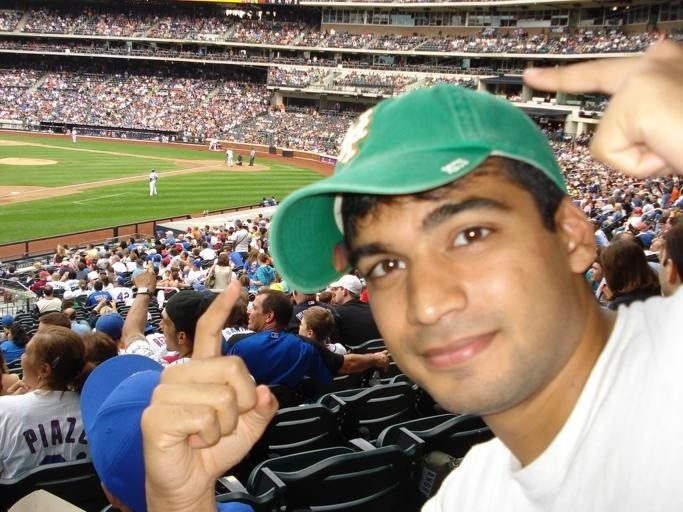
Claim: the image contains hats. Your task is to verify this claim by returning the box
[154,254,161,262]
[270,282,283,292]
[135,259,143,265]
[114,276,124,285]
[330,274,362,297]
[81,354,165,512]
[96,313,125,340]
[156,230,164,235]
[2,315,13,325]
[191,260,200,267]
[79,251,87,256]
[144,320,155,334]
[270,85,568,294]
[165,231,173,236]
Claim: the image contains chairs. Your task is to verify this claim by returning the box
[380,372,430,408]
[0,459,111,512]
[333,366,383,392]
[383,362,402,379]
[228,405,339,483]
[318,384,419,438]
[266,376,319,410]
[375,413,495,512]
[215,436,406,512]
[0,269,196,381]
[343,339,388,354]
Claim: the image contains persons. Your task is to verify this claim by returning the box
[226,149,233,166]
[555,151,683,246]
[236,153,243,166]
[122,260,208,370]
[537,119,596,151]
[38,312,72,330]
[438,34,487,90]
[492,66,614,113]
[73,6,140,125]
[95,314,126,355]
[1,316,14,341]
[342,29,375,153]
[1,323,32,372]
[329,275,374,355]
[64,308,91,330]
[140,9,341,45]
[149,169,158,197]
[0,237,139,312]
[209,139,218,150]
[281,47,308,151]
[285,288,345,335]
[259,198,270,207]
[310,45,342,152]
[139,42,682,510]
[139,213,368,301]
[72,347,259,510]
[210,45,247,140]
[657,226,683,299]
[269,196,278,205]
[249,42,280,147]
[376,33,436,97]
[248,148,255,166]
[1,6,72,133]
[489,29,681,56]
[71,330,118,396]
[0,324,100,484]
[180,45,209,145]
[597,235,657,308]
[89,299,118,330]
[141,45,178,142]
[299,306,346,355]
[72,127,77,143]
[228,289,390,385]
[222,291,257,347]
